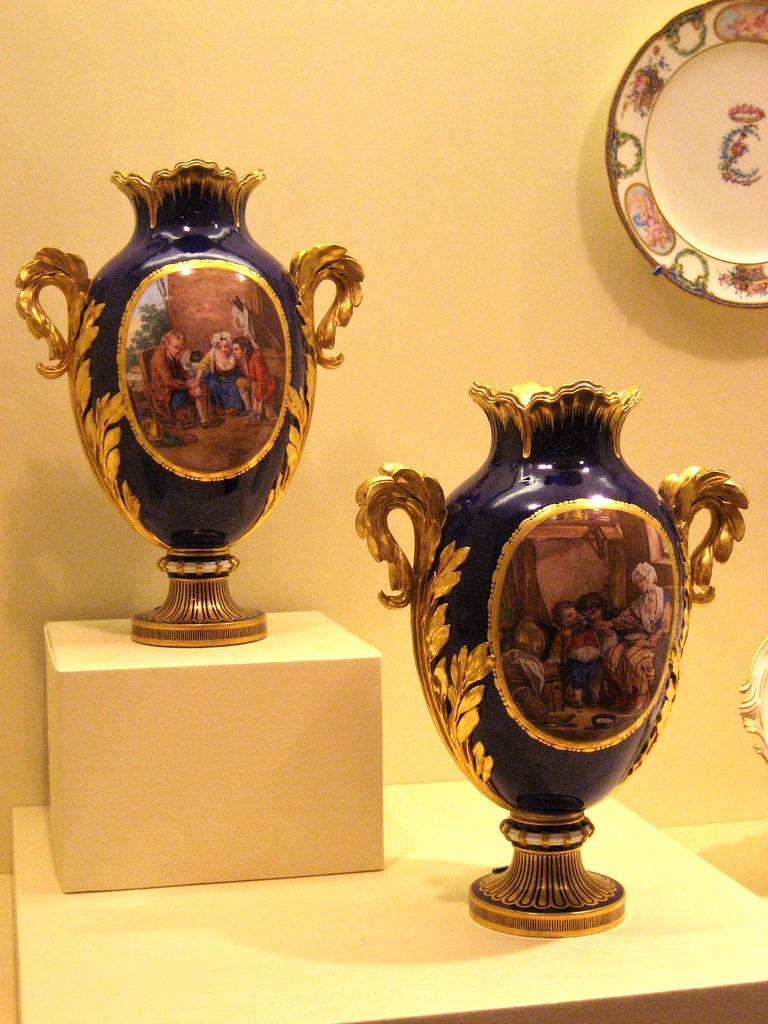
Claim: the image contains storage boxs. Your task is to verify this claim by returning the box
[42,610,383,891]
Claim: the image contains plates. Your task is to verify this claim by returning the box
[604,0,768,314]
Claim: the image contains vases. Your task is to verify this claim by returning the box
[353,378,749,939]
[15,158,365,649]
